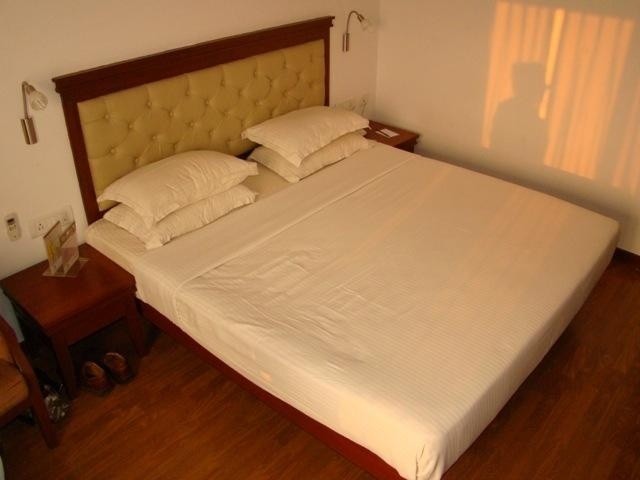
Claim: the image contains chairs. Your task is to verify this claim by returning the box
[0,316,61,452]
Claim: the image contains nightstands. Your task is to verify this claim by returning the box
[0,243,149,400]
[363,120,420,154]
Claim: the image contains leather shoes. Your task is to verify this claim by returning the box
[98,352,129,383]
[77,361,112,393]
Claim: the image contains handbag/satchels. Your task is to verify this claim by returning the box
[20,368,72,424]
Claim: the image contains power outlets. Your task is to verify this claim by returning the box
[27,215,51,239]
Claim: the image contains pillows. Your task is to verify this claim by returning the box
[241,104,369,165]
[96,149,260,229]
[103,183,259,251]
[247,133,374,185]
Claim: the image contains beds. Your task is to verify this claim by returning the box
[51,14,620,479]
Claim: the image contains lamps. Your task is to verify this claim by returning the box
[342,10,374,53]
[20,80,49,146]
